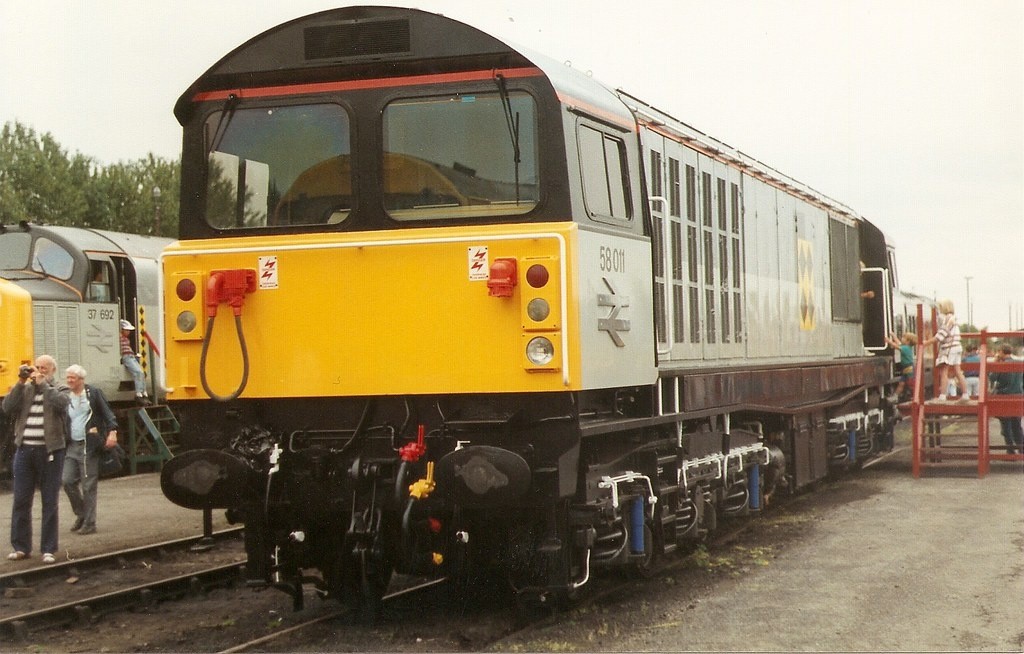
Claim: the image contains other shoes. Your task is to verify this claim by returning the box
[134,394,152,407]
[70,518,96,535]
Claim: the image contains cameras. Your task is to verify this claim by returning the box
[19,365,34,378]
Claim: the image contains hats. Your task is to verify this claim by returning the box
[120,318,135,331]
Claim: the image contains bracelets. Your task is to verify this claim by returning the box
[114,430,117,434]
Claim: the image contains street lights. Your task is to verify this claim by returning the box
[965,276,973,345]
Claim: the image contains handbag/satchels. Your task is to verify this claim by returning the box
[936,354,948,367]
[100,444,126,476]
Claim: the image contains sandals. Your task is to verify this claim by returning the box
[7,550,29,559]
[42,551,56,563]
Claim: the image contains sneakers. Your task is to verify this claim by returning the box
[928,397,946,406]
[955,398,971,406]
[887,395,899,404]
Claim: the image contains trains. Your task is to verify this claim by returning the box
[157,4,905,612]
[0,220,179,480]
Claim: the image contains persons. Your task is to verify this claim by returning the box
[118,318,154,407]
[62,365,118,535]
[1,356,70,563]
[923,298,970,403]
[859,260,876,357]
[85,266,110,302]
[885,328,920,402]
[963,341,1024,456]
